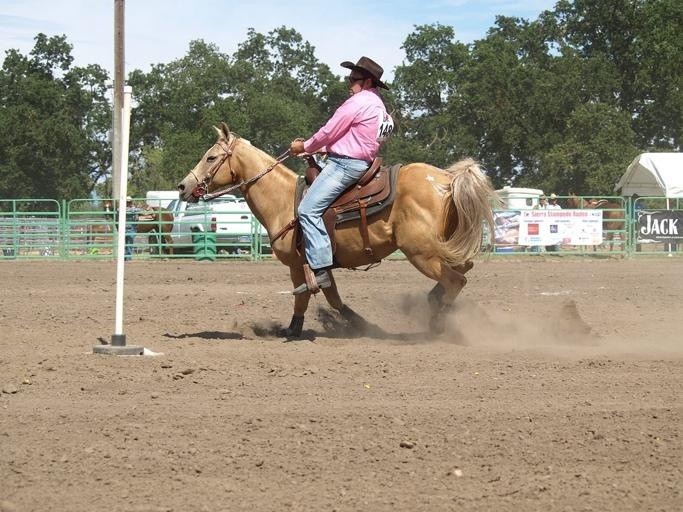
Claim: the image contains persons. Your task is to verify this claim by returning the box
[626,193,645,255]
[494,214,521,226]
[115,195,144,261]
[288,57,394,295]
[545,193,564,257]
[532,194,547,256]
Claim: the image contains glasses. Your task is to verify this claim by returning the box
[347,75,370,84]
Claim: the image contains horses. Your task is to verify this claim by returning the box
[177,121,509,342]
[561,192,625,260]
[102,197,174,259]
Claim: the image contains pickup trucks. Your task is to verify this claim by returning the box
[147,194,269,255]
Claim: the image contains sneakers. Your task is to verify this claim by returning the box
[291,268,332,296]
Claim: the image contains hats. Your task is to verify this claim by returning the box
[340,55,391,92]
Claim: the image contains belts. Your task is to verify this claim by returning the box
[326,151,358,161]
